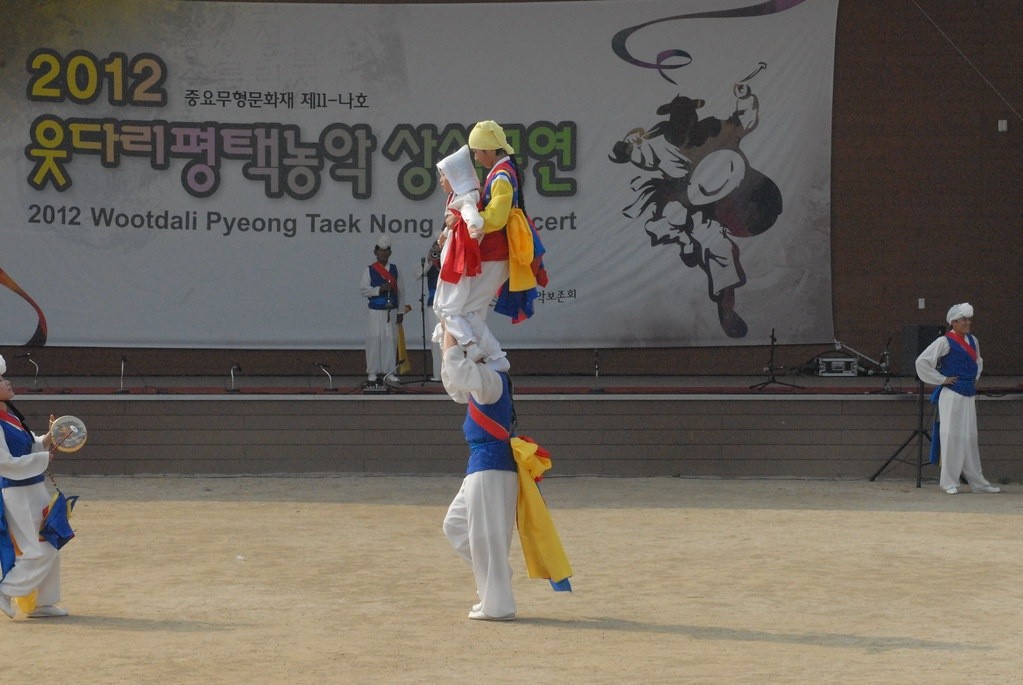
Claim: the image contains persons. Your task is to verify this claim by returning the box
[435,144,484,363]
[359,237,405,383]
[435,121,527,373]
[915,302,1001,494]
[431,320,521,622]
[0,357,78,619]
[425,239,462,382]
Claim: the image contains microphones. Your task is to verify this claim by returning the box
[421,257,425,266]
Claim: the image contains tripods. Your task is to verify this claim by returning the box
[750,328,806,391]
[870,377,967,489]
[400,266,441,386]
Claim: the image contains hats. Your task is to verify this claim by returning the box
[947,302,973,324]
[469,121,515,156]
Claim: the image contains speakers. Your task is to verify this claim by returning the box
[899,324,946,377]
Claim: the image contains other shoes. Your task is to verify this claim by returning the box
[27,605,69,617]
[0,594,15,618]
[946,487,958,493]
[386,373,400,380]
[472,601,484,612]
[468,610,515,620]
[972,485,1000,493]
[368,373,377,381]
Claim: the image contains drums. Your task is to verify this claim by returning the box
[51,415,88,453]
